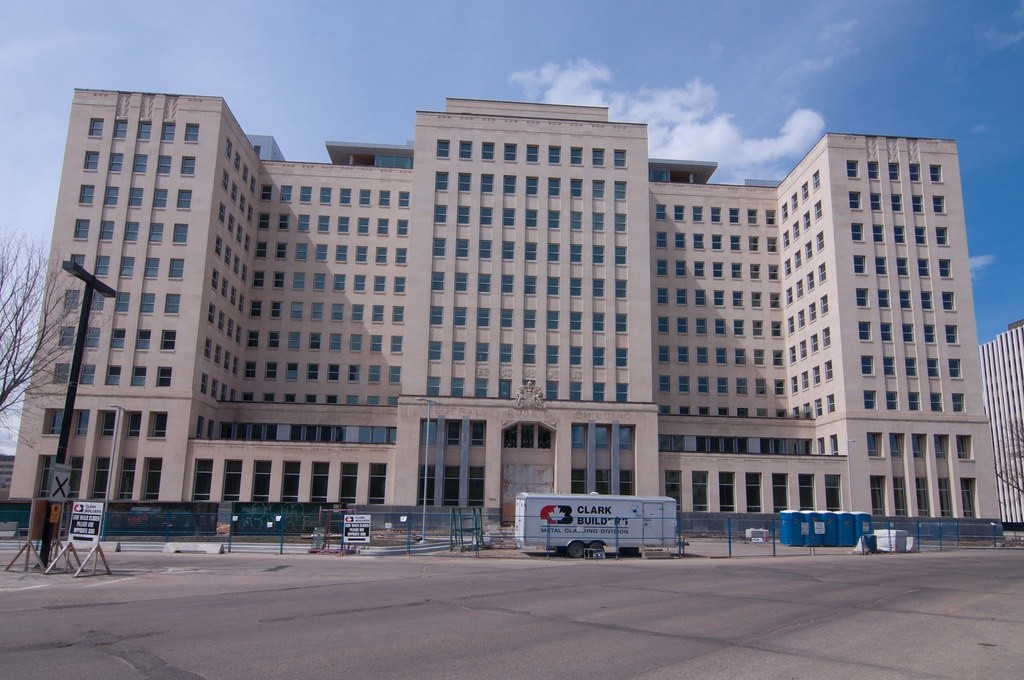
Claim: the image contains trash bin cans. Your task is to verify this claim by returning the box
[864,534,877,554]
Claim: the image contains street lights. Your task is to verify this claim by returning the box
[34,259,119,568]
